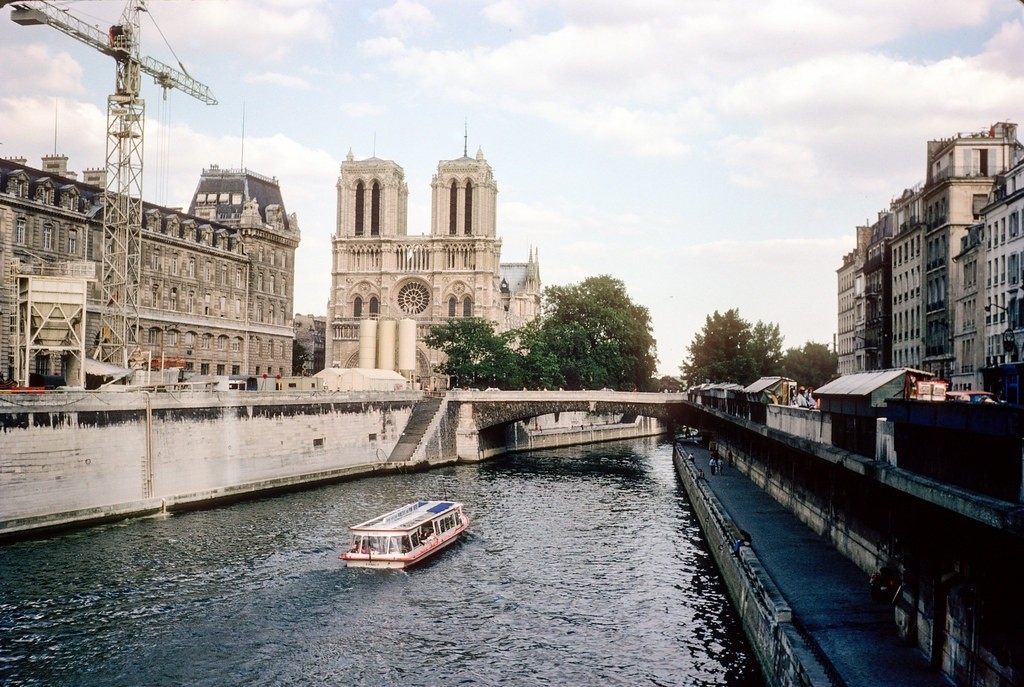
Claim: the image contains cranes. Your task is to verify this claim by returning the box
[1,1,221,373]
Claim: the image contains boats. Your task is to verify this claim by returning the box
[338,497,472,574]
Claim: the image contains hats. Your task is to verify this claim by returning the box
[798,386,805,391]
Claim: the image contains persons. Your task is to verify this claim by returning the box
[730,529,752,557]
[688,452,695,464]
[352,525,445,553]
[422,383,686,394]
[708,449,724,476]
[696,468,710,482]
[876,566,902,603]
[790,385,821,410]
